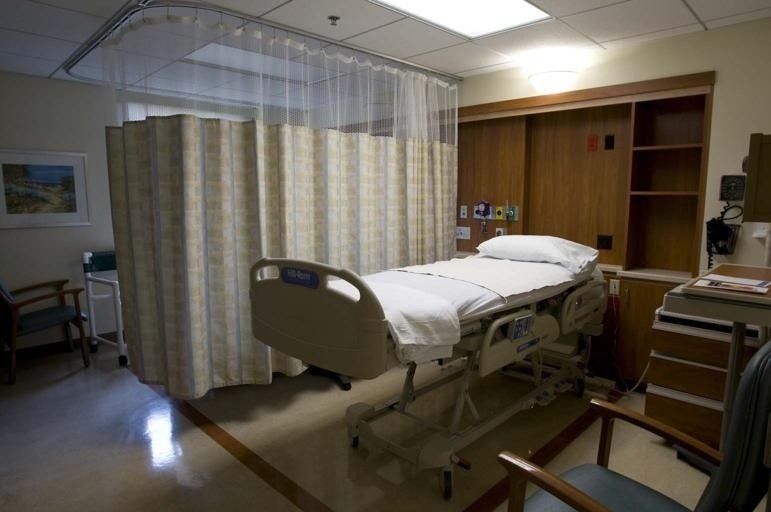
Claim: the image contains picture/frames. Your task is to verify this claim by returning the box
[0,148,93,228]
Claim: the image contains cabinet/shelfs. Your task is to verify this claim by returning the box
[642,261,768,448]
[457,70,716,393]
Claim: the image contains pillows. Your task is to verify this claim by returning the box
[476,234,599,273]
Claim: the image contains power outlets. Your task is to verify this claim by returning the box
[495,228,504,237]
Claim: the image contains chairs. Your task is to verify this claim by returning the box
[0,279,90,385]
[497,335,771,511]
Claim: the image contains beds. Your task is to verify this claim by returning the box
[249,235,611,501]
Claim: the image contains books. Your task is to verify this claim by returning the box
[704,280,770,295]
[693,278,710,287]
[702,273,771,288]
